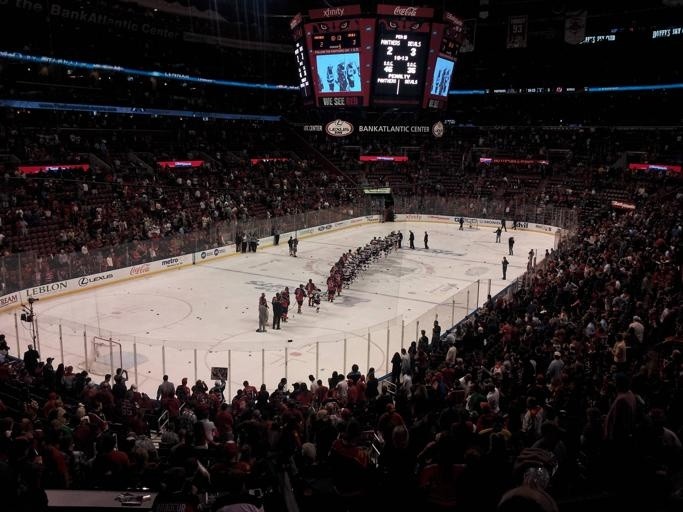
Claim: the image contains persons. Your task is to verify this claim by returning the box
[1,109,683,512]
[443,69,449,92]
[437,68,446,96]
[433,70,442,94]
[335,59,347,92]
[345,61,354,88]
[325,64,334,92]
